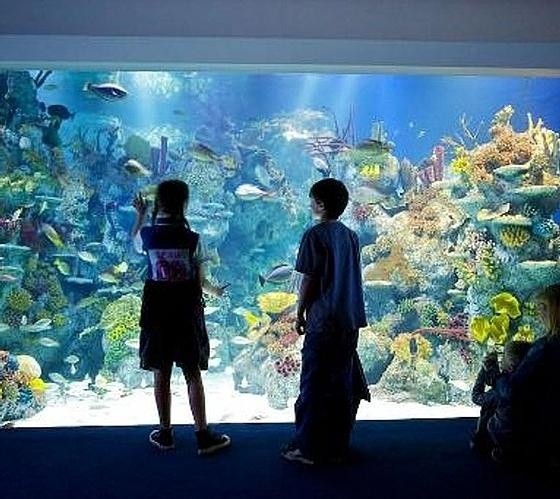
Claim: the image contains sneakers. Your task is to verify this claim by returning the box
[192,425,233,457]
[148,426,176,450]
[278,441,317,466]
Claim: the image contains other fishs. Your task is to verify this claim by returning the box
[0,101,299,393]
[80,80,129,102]
[42,83,60,91]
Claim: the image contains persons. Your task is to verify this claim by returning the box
[131,179,231,455]
[280,177,368,470]
[470,283,560,498]
